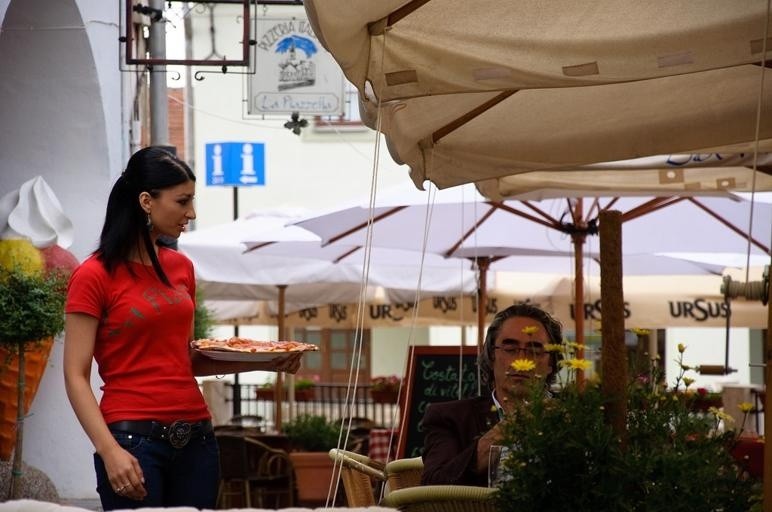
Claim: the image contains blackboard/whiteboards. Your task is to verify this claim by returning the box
[395,346,496,460]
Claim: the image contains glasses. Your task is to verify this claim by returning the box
[494,343,554,358]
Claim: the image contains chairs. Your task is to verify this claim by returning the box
[329,448,500,512]
[214,434,295,508]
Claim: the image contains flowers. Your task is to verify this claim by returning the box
[372,376,402,391]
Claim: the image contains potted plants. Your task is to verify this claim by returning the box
[254,378,314,401]
[281,413,347,501]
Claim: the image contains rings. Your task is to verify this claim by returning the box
[118,483,130,492]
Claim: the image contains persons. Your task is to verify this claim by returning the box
[422,305,565,488]
[64,147,300,512]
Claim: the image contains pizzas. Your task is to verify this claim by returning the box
[190,338,318,352]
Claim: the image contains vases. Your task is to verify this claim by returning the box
[371,390,398,404]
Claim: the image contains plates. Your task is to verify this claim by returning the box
[195,348,291,363]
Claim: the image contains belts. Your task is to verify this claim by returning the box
[108,421,200,449]
